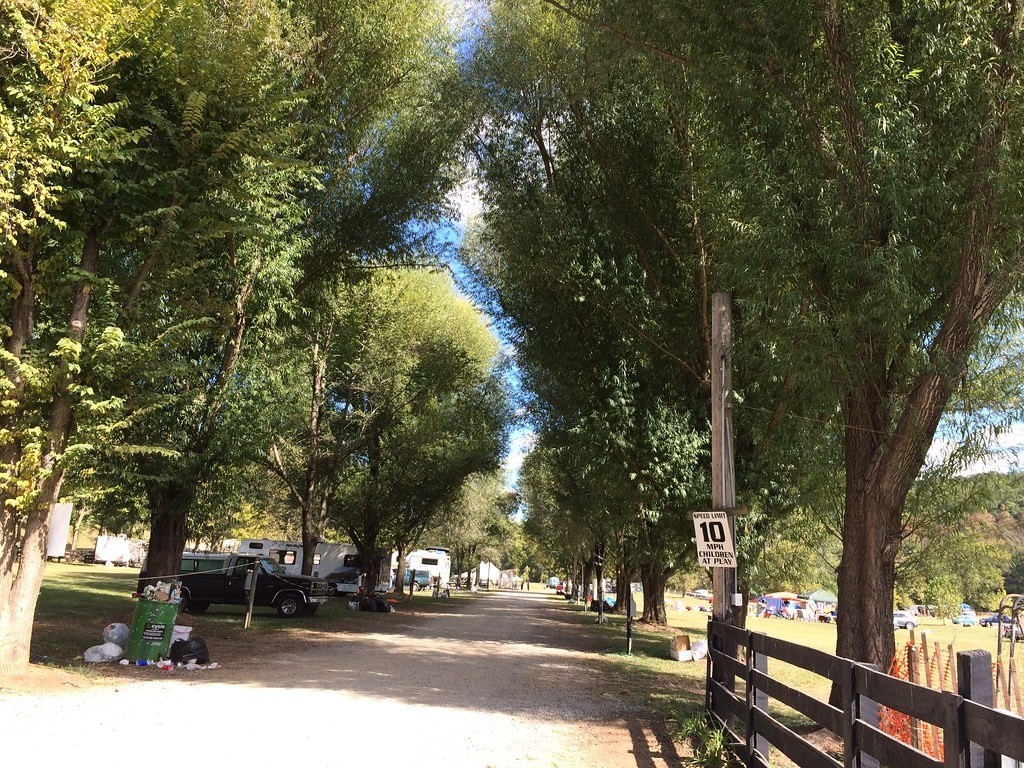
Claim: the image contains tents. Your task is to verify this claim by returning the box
[765,588,838,614]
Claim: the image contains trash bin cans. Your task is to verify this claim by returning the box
[122,596,181,662]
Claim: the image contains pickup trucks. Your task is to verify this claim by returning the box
[132,550,330,618]
[392,569,431,591]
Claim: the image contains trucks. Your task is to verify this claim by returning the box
[233,536,393,596]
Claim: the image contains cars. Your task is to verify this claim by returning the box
[952,614,977,627]
[1003,624,1024,642]
[980,614,1013,629]
[892,610,918,630]
[961,602,972,614]
